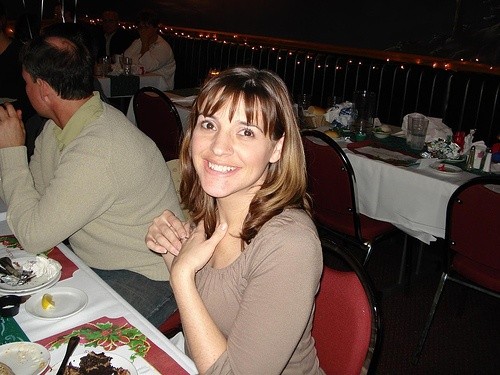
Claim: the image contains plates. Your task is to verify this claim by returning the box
[45,352,138,375]
[440,158,465,163]
[0,341,51,375]
[25,286,89,320]
[0,256,61,296]
[428,163,464,175]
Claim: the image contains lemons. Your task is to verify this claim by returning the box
[42,294,55,311]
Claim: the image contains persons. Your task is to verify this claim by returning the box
[0,0,176,96]
[0,37,189,330]
[145,68,326,375]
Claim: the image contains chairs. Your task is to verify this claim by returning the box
[300,130,399,267]
[133,85,183,161]
[410,174,500,363]
[312,237,381,375]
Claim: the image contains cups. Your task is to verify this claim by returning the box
[95,57,112,77]
[121,57,132,74]
[407,115,429,152]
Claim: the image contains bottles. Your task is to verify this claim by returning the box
[463,146,475,171]
[482,148,492,172]
[301,94,309,109]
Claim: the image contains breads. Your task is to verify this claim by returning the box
[307,105,326,115]
[325,130,337,138]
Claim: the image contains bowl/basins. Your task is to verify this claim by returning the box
[372,131,390,139]
[0,294,20,317]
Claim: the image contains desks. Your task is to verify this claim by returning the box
[126,87,500,293]
[0,211,199,375]
[99,73,168,98]
